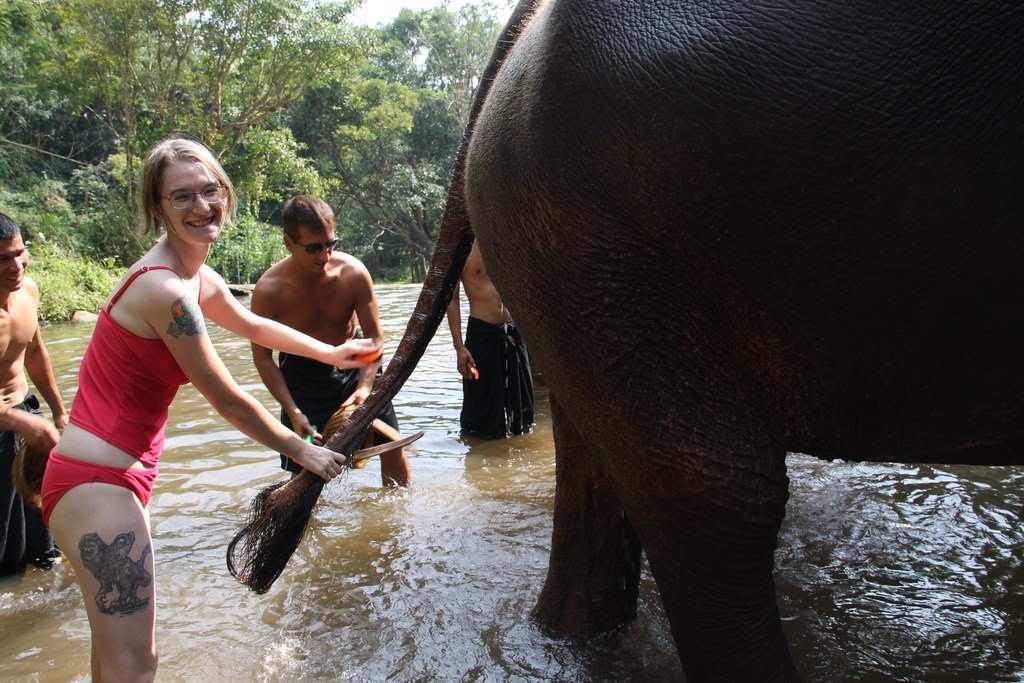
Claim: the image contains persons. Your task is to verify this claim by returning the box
[0,209,70,578]
[250,194,411,488]
[445,236,535,443]
[41,138,385,683]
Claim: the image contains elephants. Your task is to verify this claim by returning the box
[225,0,1024,683]
